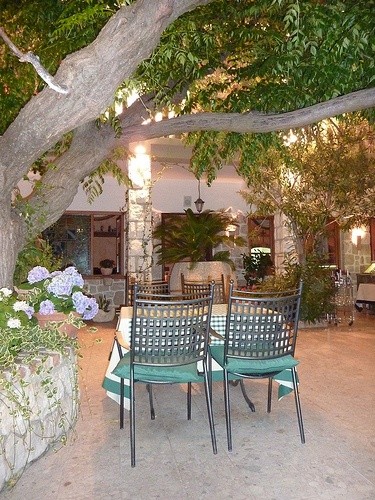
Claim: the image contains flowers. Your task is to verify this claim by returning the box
[0,266,99,352]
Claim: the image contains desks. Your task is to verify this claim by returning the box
[102,303,300,411]
[354,272,375,312]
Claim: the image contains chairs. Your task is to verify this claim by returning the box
[203,279,305,452]
[125,272,226,307]
[111,280,217,468]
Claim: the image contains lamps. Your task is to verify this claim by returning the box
[194,199,205,213]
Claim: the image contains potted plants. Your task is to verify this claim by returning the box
[100,259,115,275]
[150,207,247,304]
